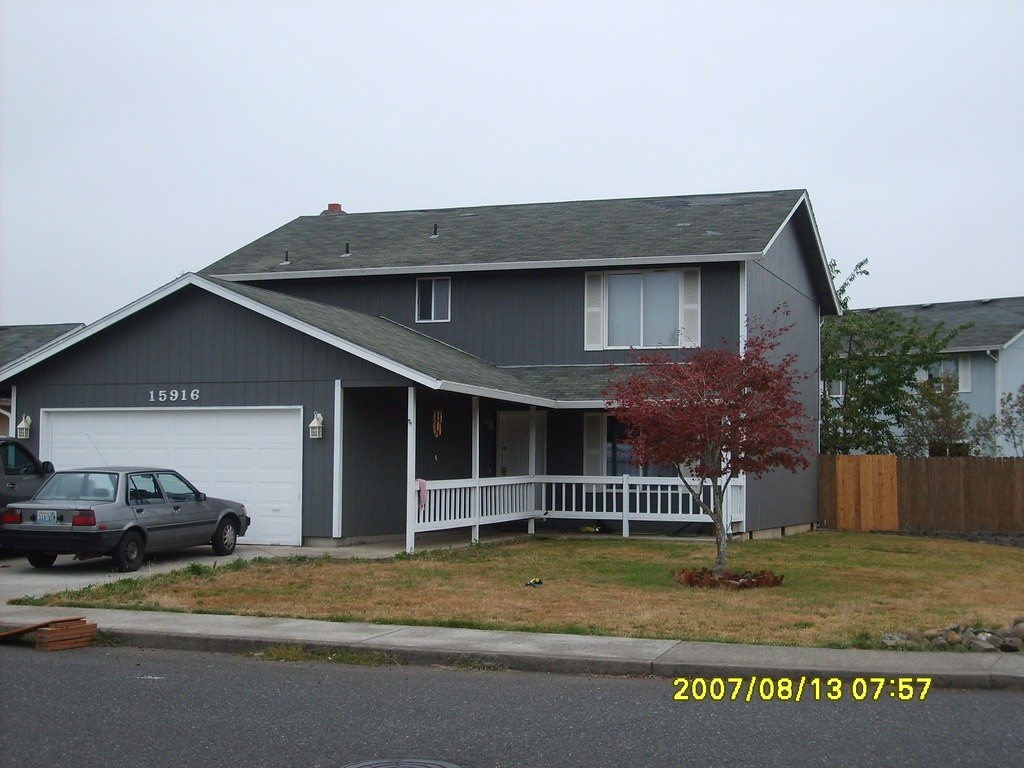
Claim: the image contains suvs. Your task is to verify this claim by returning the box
[0,435,94,558]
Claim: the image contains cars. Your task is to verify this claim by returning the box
[1,467,252,572]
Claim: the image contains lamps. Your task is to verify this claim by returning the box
[307,411,324,439]
[17,413,32,439]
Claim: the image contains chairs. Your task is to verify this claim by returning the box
[93,488,109,497]
